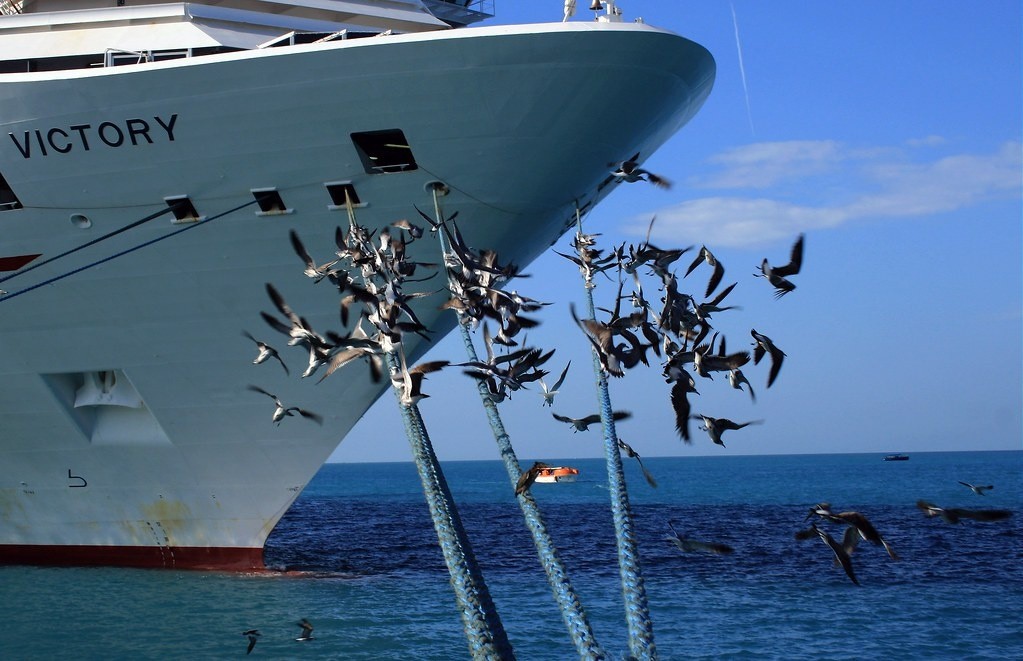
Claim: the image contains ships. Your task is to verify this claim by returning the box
[0,0,716,569]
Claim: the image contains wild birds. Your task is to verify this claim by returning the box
[237,617,313,656]
[242,153,1012,586]
